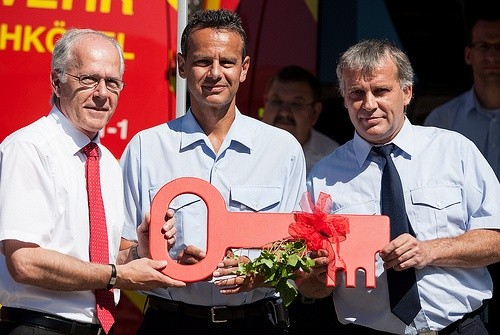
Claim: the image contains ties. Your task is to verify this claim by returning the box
[81,142,116,335]
[372,144,422,326]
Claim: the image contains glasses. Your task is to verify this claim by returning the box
[64,73,125,92]
[266,96,315,111]
[472,41,500,52]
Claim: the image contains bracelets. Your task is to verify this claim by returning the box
[131,244,140,260]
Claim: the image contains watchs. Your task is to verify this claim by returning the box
[104,264,117,291]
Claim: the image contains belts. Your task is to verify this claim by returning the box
[295,292,334,304]
[149,295,283,323]
[0,308,110,332]
[417,311,480,335]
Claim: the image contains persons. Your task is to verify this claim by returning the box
[0,28,186,335]
[423,10,500,184]
[119,7,307,335]
[306,39,500,335]
[263,65,340,180]
